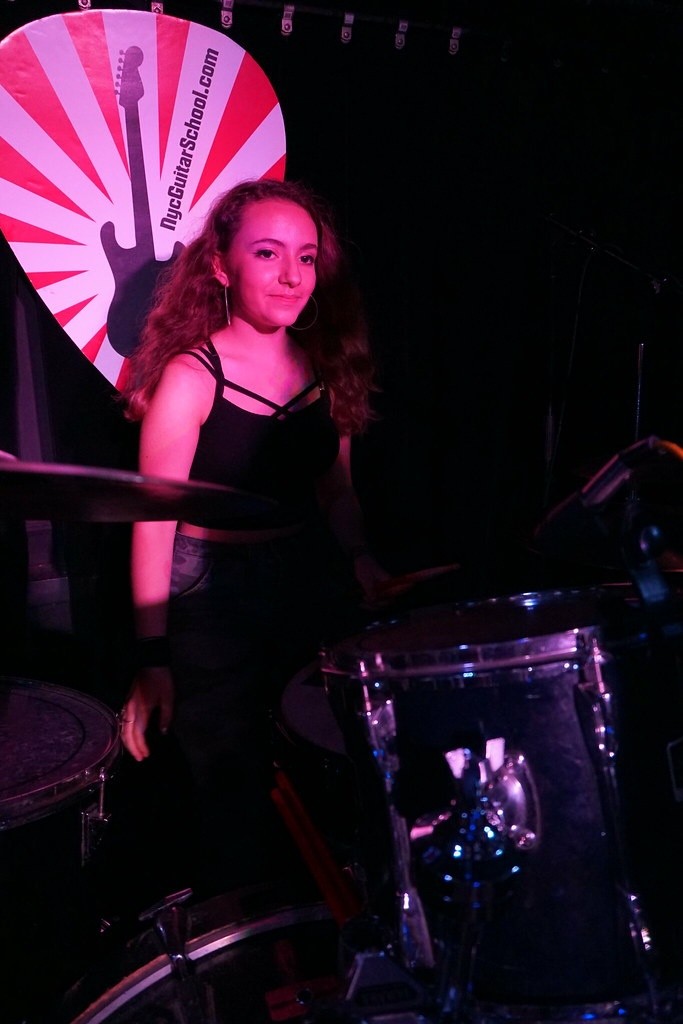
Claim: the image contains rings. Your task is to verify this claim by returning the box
[119,715,136,726]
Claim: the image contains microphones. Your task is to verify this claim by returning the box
[534,437,668,554]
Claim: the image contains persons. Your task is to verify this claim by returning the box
[118,183,372,785]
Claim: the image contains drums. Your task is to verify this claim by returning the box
[0,673,127,1023]
[61,875,411,1024]
[329,577,683,1024]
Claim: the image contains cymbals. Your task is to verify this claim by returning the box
[3,454,280,527]
